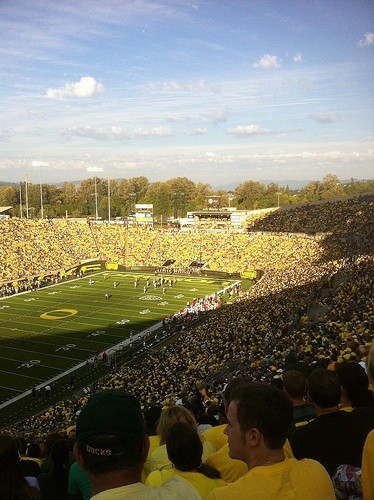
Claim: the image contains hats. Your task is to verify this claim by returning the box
[76,388,146,474]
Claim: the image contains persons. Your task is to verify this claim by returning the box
[332,427,374,500]
[0,455,95,500]
[203,383,336,500]
[201,377,295,463]
[72,390,202,500]
[204,433,294,483]
[1,192,373,455]
[288,368,371,478]
[146,423,229,500]
[147,404,215,472]
[138,405,164,485]
